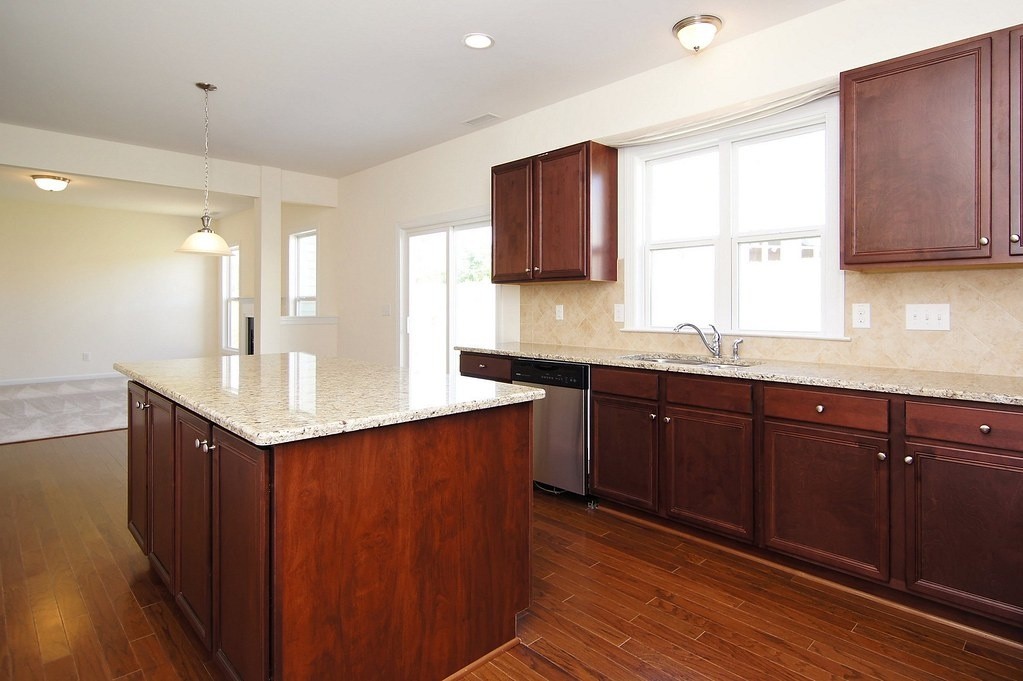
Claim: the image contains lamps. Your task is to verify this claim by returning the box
[173,82,233,255]
[672,14,722,54]
[31,174,70,191]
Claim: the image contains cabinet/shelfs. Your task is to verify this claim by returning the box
[759,379,1023,629]
[458,349,590,500]
[838,25,1023,269]
[490,139,617,286]
[589,363,758,549]
[120,349,546,681]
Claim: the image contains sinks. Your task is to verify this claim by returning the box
[621,352,768,372]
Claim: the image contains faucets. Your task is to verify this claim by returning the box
[673,322,723,358]
